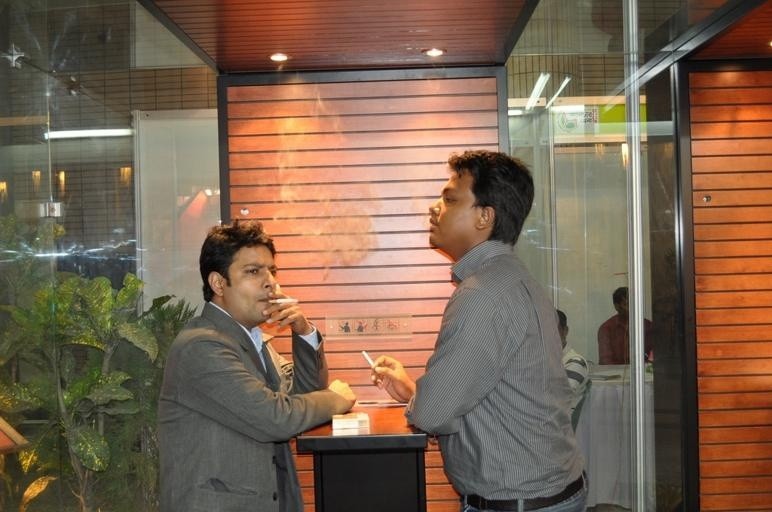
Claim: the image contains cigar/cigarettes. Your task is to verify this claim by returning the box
[362,350,377,369]
[267,298,299,304]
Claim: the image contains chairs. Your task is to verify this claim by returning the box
[572,380,594,435]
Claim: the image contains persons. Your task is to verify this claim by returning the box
[366,147,592,511]
[551,308,589,422]
[596,286,653,365]
[155,215,358,508]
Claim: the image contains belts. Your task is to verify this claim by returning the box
[458,472,589,510]
